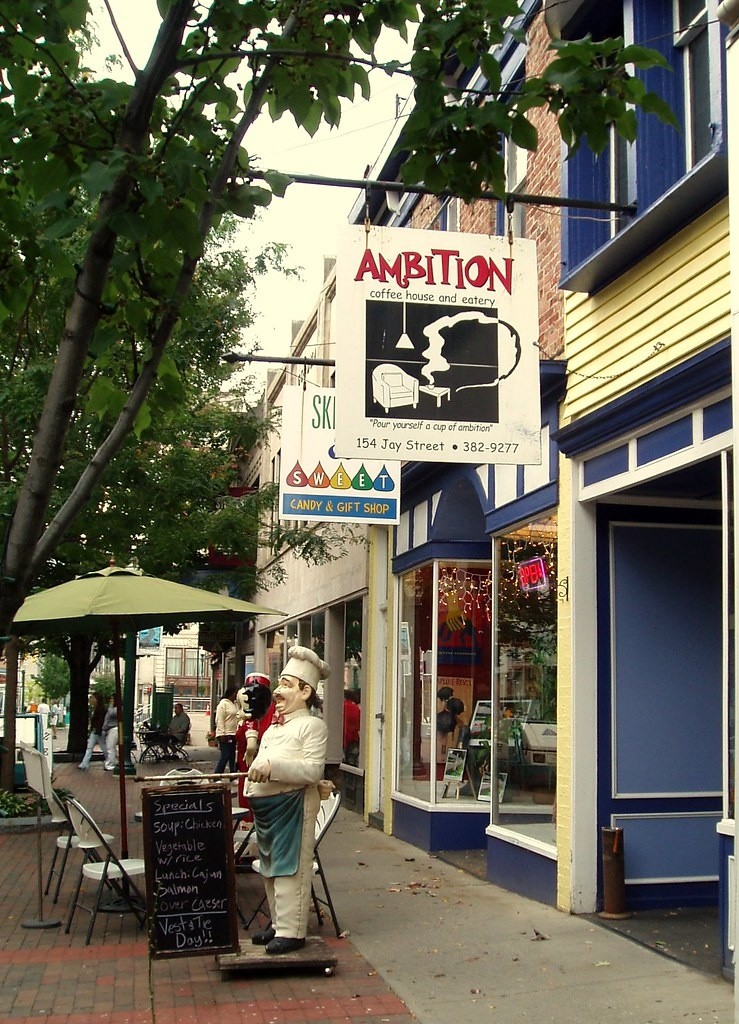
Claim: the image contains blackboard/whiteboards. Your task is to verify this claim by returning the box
[140,783,242,961]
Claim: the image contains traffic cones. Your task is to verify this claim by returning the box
[205,703,210,716]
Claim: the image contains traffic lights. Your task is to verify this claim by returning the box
[147,689,151,696]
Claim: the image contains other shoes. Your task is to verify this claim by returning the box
[170,755,179,760]
[162,753,171,760]
[52,735,57,739]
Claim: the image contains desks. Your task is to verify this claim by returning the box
[132,806,249,932]
[136,731,160,764]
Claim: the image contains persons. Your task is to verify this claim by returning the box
[236,672,277,824]
[243,644,331,956]
[37,698,50,736]
[78,693,108,769]
[160,703,191,760]
[101,694,119,771]
[49,699,60,738]
[213,685,239,783]
[26,701,38,713]
[343,687,361,768]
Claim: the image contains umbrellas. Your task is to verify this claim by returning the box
[13,563,288,895]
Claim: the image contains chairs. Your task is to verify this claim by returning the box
[43,787,122,905]
[243,787,364,940]
[167,722,193,765]
[233,825,255,864]
[159,767,209,786]
[61,796,147,946]
